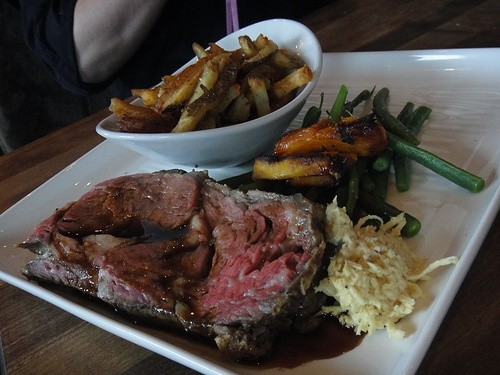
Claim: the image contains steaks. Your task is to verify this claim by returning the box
[14,169,335,365]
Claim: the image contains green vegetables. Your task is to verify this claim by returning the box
[217,81,485,238]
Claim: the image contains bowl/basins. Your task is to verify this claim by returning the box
[96,19,323,168]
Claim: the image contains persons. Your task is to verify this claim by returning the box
[1,1,167,157]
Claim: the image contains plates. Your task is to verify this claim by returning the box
[0,49,499,375]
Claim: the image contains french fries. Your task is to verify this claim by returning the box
[108,33,313,132]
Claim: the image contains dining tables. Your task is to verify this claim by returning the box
[0,3,500,374]
